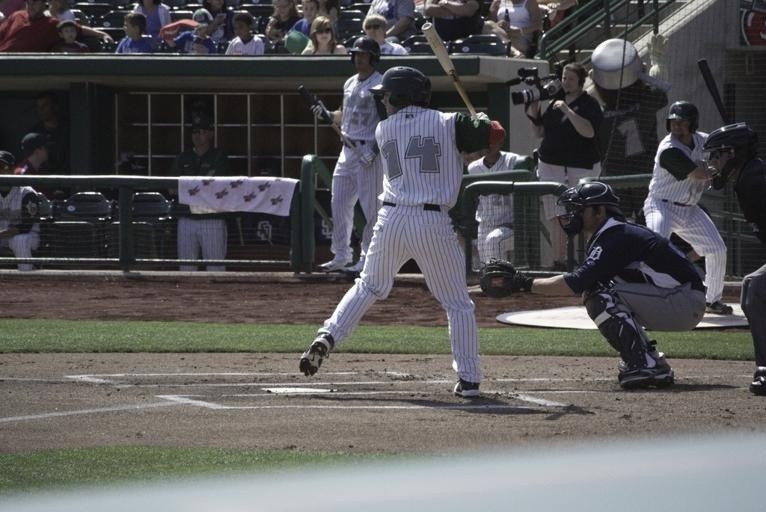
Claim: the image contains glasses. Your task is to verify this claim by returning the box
[365,23,380,30]
[317,26,330,34]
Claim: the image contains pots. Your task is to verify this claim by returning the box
[590,37,673,91]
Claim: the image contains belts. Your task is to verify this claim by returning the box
[381,201,442,212]
[343,140,366,149]
[661,198,694,207]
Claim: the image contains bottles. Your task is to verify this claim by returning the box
[503,7,511,32]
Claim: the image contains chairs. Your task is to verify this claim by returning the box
[0,0,534,272]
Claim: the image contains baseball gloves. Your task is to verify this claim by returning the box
[479,258,520,298]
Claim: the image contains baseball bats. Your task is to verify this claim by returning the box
[420,21,476,115]
[698,59,729,124]
[297,84,363,158]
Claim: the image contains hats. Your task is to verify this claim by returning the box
[58,12,78,27]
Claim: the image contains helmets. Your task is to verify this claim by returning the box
[555,181,619,236]
[368,66,432,108]
[348,38,381,64]
[666,99,698,132]
[702,123,756,190]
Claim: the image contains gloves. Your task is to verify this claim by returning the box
[359,149,377,168]
[311,100,335,122]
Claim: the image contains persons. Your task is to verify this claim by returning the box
[299,65,504,398]
[480,180,706,388]
[597,72,672,224]
[0,0,577,274]
[524,62,614,271]
[639,101,733,316]
[702,122,765,394]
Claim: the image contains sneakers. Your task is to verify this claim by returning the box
[453,378,480,397]
[616,354,675,388]
[706,301,734,315]
[299,331,335,378]
